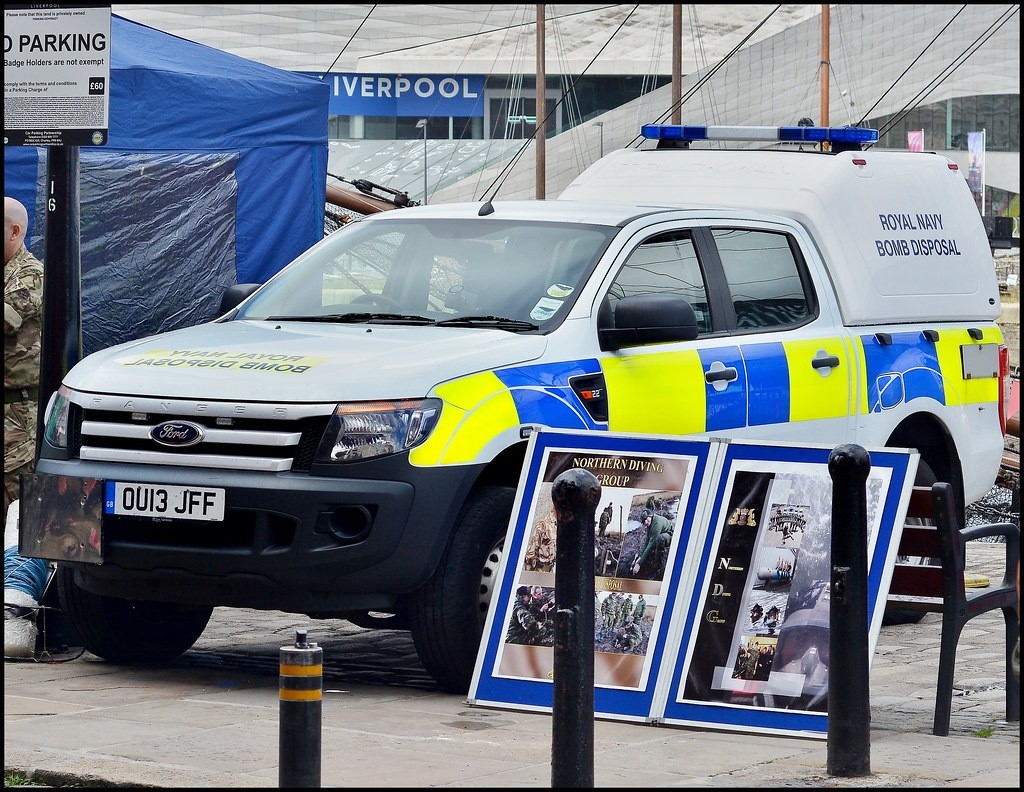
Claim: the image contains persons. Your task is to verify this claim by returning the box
[969,153,981,188]
[732,641,775,680]
[630,510,674,576]
[525,505,557,573]
[775,505,804,518]
[776,556,792,581]
[505,585,555,646]
[2,197,44,518]
[594,591,646,652]
[598,502,613,536]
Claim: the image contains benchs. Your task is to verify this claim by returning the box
[886,481,1022,736]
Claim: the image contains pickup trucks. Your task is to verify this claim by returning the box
[17,127,1008,696]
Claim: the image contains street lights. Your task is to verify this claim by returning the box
[593,121,603,159]
[416,119,428,207]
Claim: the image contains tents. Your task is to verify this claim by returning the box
[4,17,330,369]
